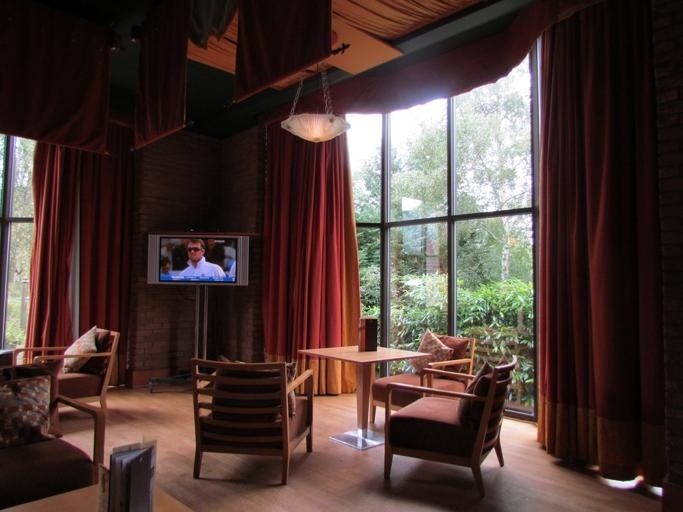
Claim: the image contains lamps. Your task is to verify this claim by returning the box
[276,62,351,147]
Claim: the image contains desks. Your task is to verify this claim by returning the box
[1,470,198,511]
[296,342,432,452]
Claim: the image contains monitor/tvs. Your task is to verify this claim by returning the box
[147,234,249,286]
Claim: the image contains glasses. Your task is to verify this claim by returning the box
[187,247,202,251]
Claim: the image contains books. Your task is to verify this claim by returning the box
[108,446,151,512]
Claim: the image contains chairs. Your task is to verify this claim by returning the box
[368,330,476,428]
[10,323,122,435]
[381,354,520,499]
[188,353,315,488]
[0,361,107,507]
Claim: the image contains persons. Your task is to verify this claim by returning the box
[160,238,236,283]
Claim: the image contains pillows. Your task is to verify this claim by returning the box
[436,333,470,379]
[84,328,110,374]
[210,355,298,420]
[0,374,57,448]
[407,330,454,379]
[62,325,98,373]
[455,356,509,421]
